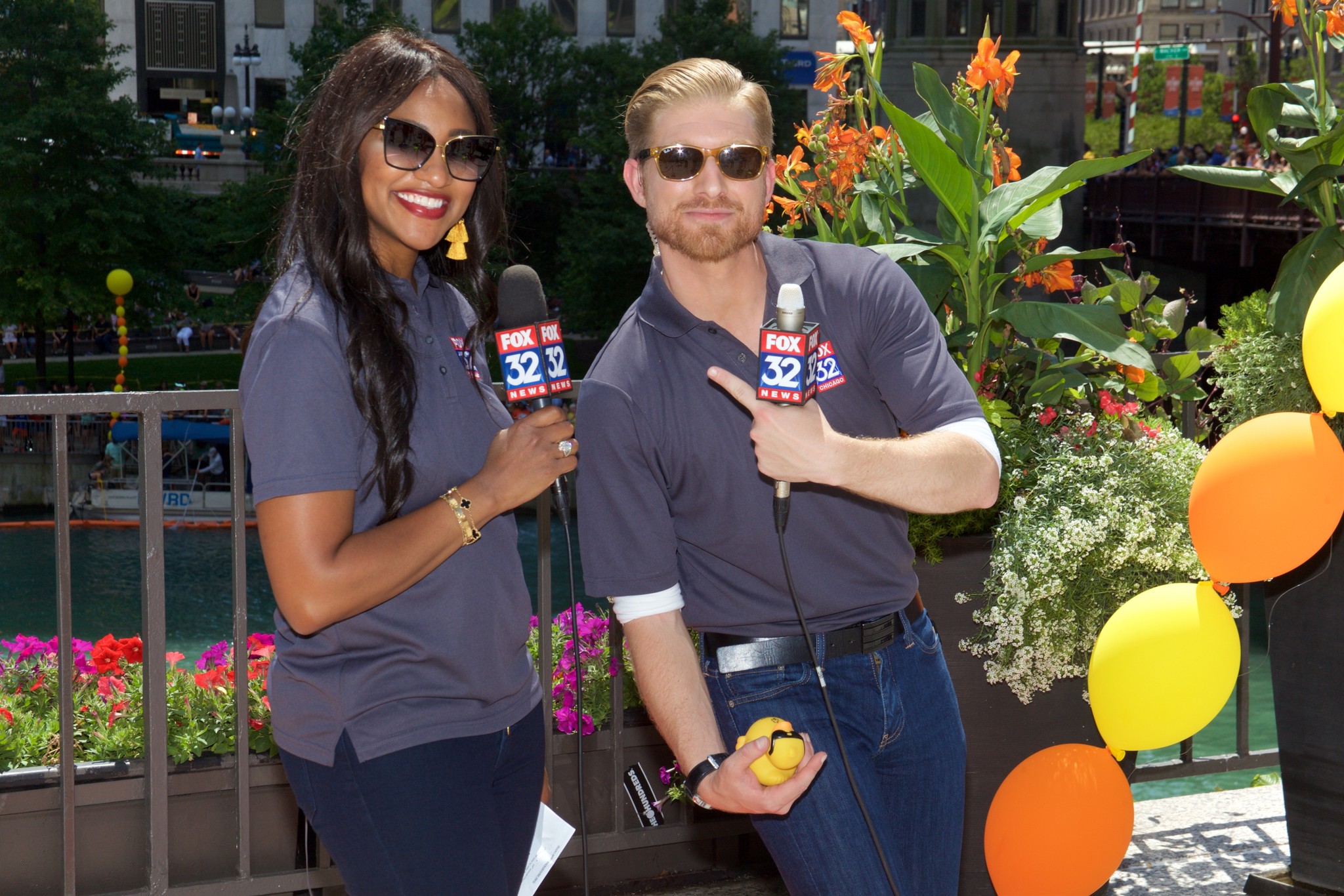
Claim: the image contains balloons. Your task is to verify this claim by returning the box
[1301,261,1344,414]
[1087,581,1242,762]
[983,744,1135,896]
[106,268,134,429]
[1187,413,1344,597]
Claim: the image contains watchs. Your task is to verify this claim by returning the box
[683,752,732,812]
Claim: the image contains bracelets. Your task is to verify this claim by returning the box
[438,485,481,547]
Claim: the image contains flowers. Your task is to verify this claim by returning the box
[765,0,1344,705]
[0,596,703,768]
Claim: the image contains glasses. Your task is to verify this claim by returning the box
[372,116,500,183]
[636,144,766,182]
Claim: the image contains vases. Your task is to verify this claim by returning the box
[0,724,758,896]
[912,538,1107,896]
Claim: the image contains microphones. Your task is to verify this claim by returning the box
[496,262,577,519]
[759,284,819,528]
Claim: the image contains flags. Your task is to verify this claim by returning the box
[1084,82,1096,114]
[1101,82,1117,118]
[1186,65,1205,116]
[1218,81,1235,122]
[1162,67,1182,117]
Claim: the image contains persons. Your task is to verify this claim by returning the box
[193,142,204,180]
[1083,139,1295,226]
[577,54,1002,895]
[0,377,230,492]
[237,24,576,896]
[163,304,240,353]
[186,280,201,304]
[226,254,263,286]
[2,312,116,359]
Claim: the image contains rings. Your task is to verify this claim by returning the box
[558,439,572,458]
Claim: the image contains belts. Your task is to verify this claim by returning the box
[705,594,928,673]
